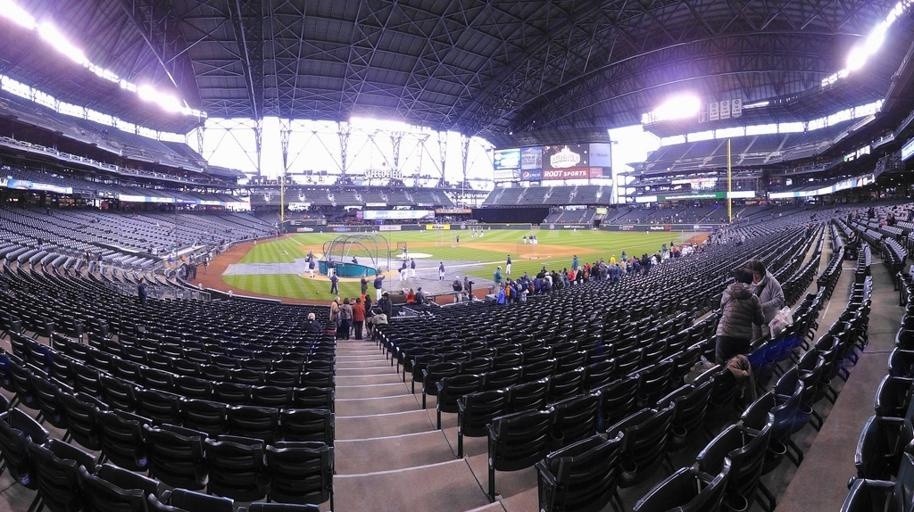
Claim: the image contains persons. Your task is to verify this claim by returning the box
[714,260,785,365]
[735,139,913,229]
[1,137,746,341]
